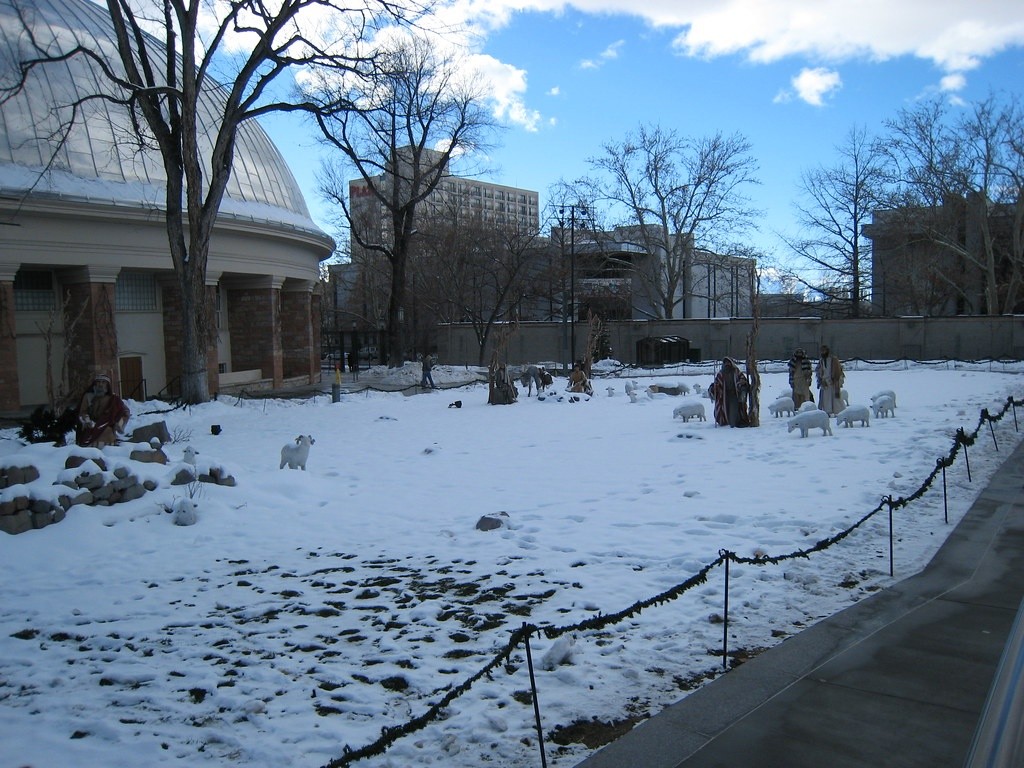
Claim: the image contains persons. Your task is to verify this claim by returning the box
[421,352,436,388]
[76,374,130,447]
[707,357,751,428]
[566,364,591,395]
[815,344,845,418]
[787,347,812,411]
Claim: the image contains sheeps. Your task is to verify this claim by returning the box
[606,380,709,423]
[280,435,315,470]
[769,388,896,438]
